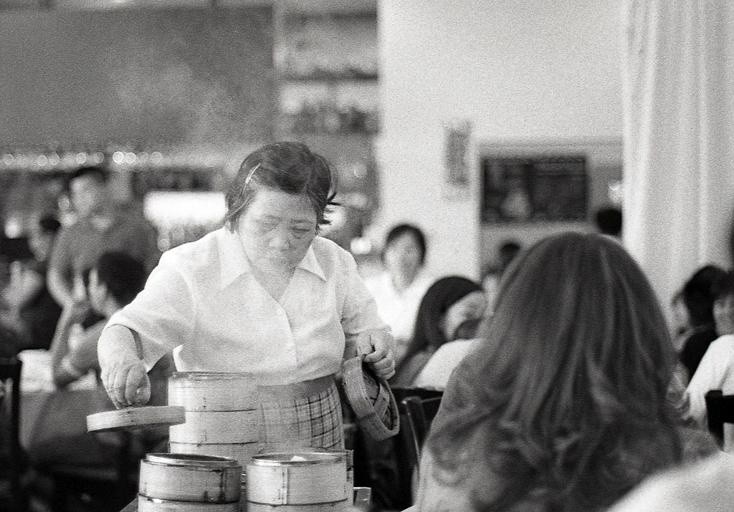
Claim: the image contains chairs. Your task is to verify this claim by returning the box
[705,390,732,451]
[401,396,441,469]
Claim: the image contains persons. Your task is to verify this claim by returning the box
[595,205,621,245]
[366,224,523,391]
[1,170,176,511]
[413,231,733,511]
[97,138,399,449]
